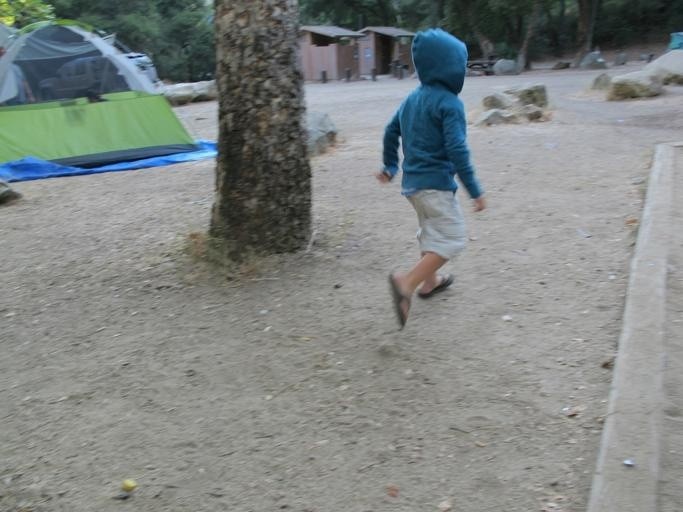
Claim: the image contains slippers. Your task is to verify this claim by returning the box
[417,271,454,298]
[389,271,410,331]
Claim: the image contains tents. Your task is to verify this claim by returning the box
[0,20,201,169]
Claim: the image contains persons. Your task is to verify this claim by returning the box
[0,46,36,107]
[375,26,485,328]
